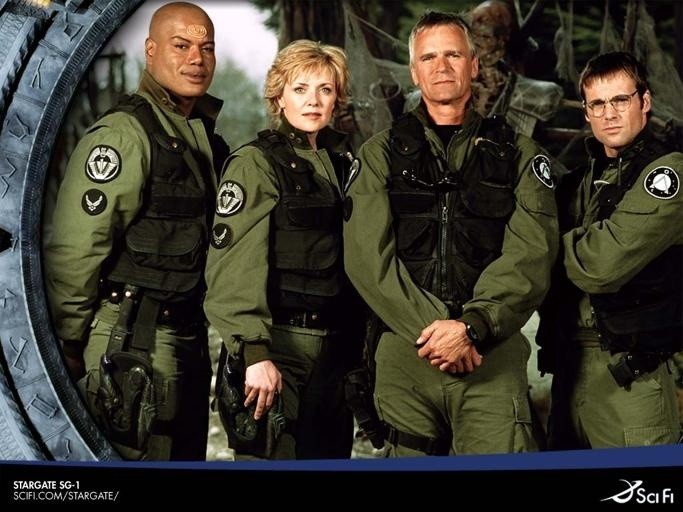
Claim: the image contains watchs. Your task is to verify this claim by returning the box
[464,322,478,342]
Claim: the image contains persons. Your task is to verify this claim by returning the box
[528,50,681,452]
[336,7,559,465]
[197,34,361,461]
[37,0,235,463]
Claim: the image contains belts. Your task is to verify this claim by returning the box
[269,304,333,331]
[96,280,192,328]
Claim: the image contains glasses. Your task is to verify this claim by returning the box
[580,89,640,119]
